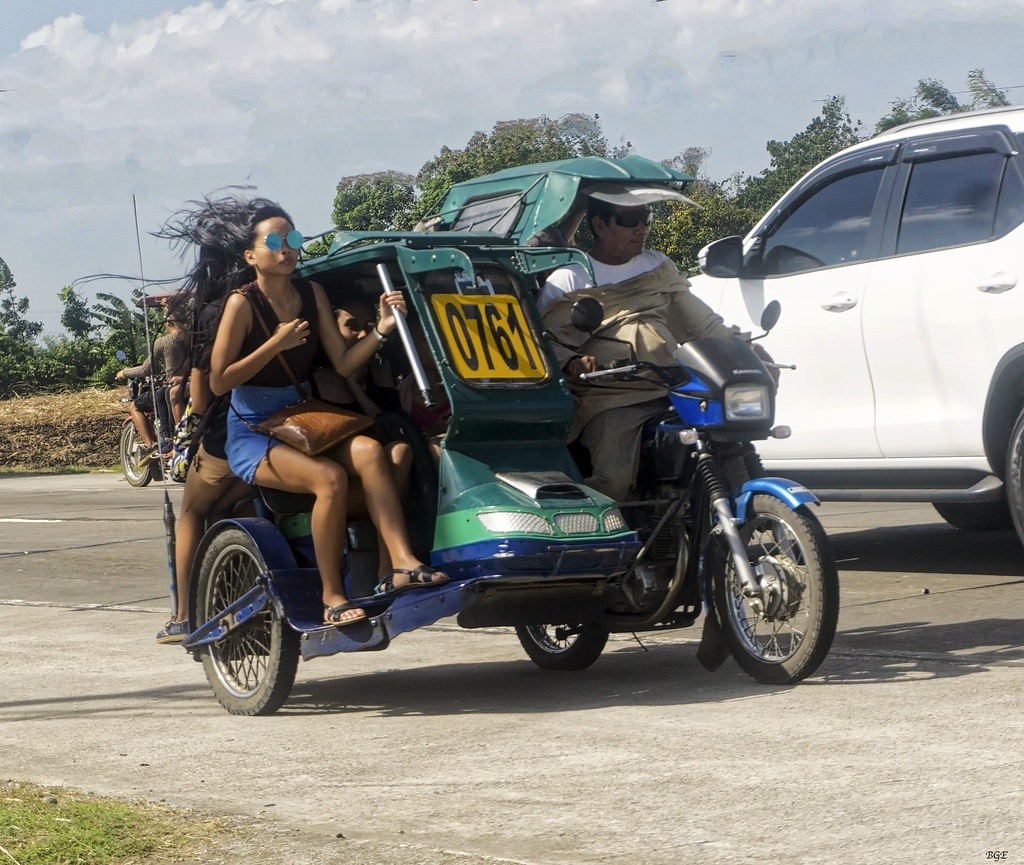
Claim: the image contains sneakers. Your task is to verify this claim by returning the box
[157,619,191,643]
[138,442,159,467]
[151,437,174,458]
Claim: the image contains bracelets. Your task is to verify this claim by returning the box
[371,325,388,343]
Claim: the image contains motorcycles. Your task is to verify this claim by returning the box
[162,154,842,716]
[114,289,201,488]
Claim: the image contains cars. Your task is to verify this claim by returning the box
[677,105,1024,569]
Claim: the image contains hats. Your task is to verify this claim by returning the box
[165,306,191,330]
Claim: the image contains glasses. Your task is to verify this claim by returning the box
[601,210,653,229]
[253,229,304,251]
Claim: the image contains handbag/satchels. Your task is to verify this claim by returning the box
[400,367,450,428]
[170,396,206,484]
[211,288,374,456]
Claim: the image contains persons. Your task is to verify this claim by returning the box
[114,302,192,468]
[315,297,451,428]
[163,201,450,625]
[154,249,229,645]
[535,190,781,501]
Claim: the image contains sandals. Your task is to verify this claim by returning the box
[321,598,366,627]
[391,564,450,592]
[376,570,403,597]
